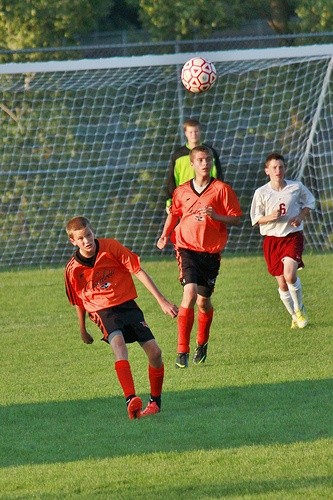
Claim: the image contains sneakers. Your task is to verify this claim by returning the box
[140,401,161,417]
[193,341,208,365]
[294,304,308,328]
[175,353,189,369]
[291,314,298,328]
[128,397,143,420]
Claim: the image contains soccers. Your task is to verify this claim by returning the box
[181,57,217,94]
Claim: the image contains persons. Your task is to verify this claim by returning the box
[64,217,178,419]
[165,118,224,218]
[250,153,316,328]
[156,145,243,369]
